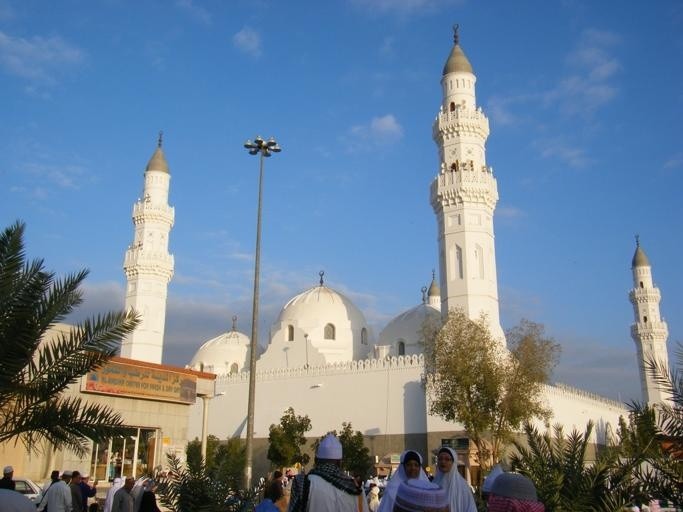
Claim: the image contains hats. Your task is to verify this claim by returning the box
[482,472,537,502]
[393,479,450,512]
[64,470,73,476]
[4,466,14,474]
[316,433,343,459]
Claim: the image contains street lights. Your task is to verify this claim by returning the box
[237,133,284,495]
[195,391,225,479]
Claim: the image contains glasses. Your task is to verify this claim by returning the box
[438,458,453,462]
[404,462,420,467]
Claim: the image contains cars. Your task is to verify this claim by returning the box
[12,478,43,504]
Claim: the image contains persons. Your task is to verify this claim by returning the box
[1,465,16,491]
[256,436,544,512]
[103,449,121,482]
[37,469,100,511]
[104,475,161,512]
